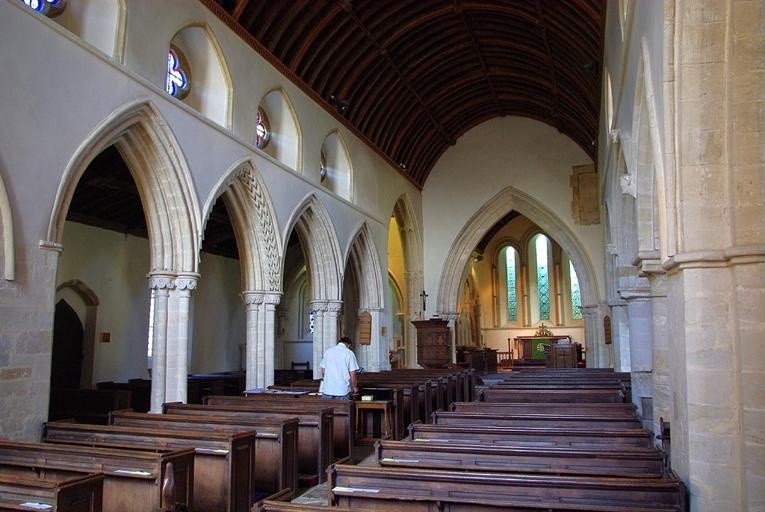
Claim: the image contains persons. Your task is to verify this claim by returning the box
[320,336,361,400]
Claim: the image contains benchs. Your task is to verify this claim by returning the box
[0,366,697,512]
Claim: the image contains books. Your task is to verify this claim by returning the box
[361,396,372,400]
[364,394,374,400]
